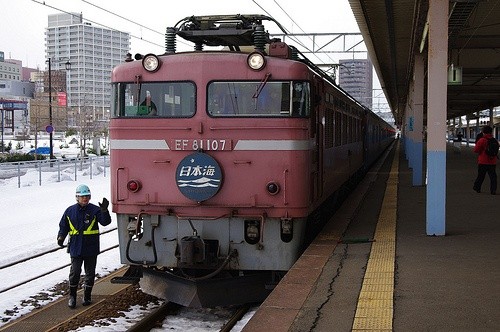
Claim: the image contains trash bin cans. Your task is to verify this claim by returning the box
[92,137,101,155]
[453,138,462,151]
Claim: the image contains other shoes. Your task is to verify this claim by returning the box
[473,186,481,193]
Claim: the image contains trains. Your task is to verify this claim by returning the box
[110,12,397,308]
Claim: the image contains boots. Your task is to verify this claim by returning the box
[82,285,93,306]
[68,284,78,308]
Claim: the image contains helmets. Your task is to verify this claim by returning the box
[76,184,91,200]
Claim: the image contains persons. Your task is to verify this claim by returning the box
[473,126,498,194]
[457,132,464,144]
[397,133,428,144]
[56,184,111,308]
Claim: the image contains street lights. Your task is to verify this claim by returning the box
[45,58,71,168]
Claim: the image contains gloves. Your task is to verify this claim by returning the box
[57,236,65,248]
[98,198,109,213]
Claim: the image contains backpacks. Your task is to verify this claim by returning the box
[484,137,499,157]
